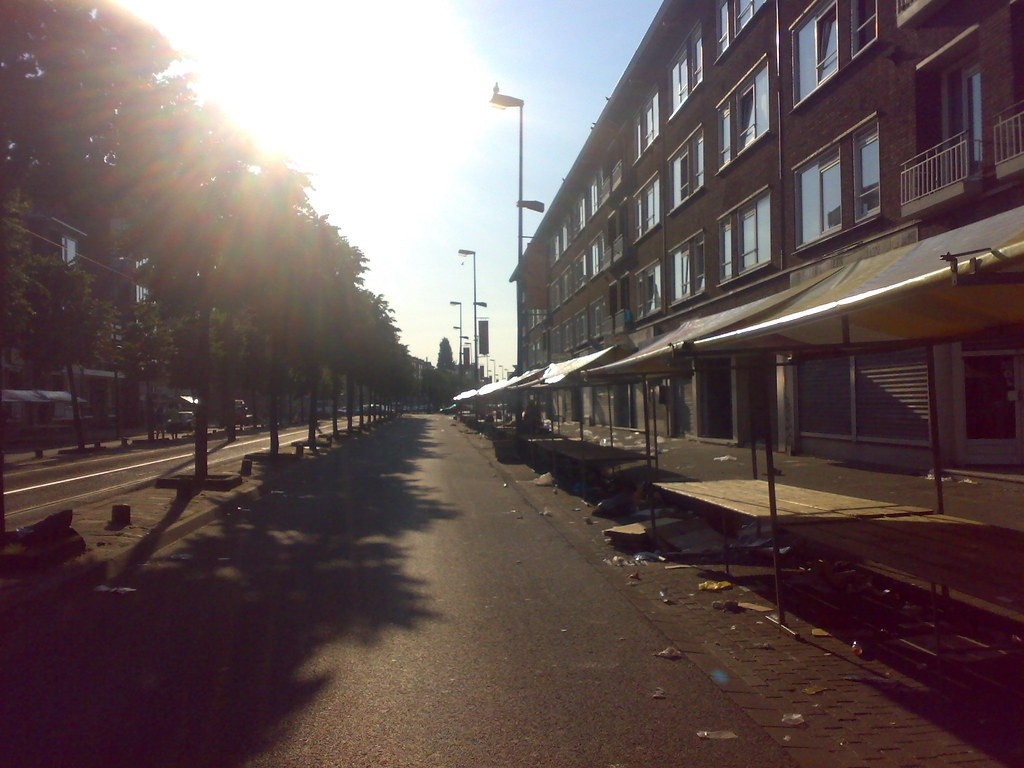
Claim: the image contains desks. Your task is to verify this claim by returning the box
[651,479,1024,687]
[461,414,654,506]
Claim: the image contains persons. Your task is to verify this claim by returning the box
[316,417,321,434]
[154,403,180,440]
[524,401,541,434]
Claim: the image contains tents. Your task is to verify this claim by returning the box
[451,204,1024,696]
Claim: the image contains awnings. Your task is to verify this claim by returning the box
[181,395,197,404]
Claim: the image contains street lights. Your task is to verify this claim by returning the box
[465,342,472,365]
[489,92,523,453]
[498,365,507,382]
[457,249,487,426]
[490,359,498,384]
[450,301,469,422]
